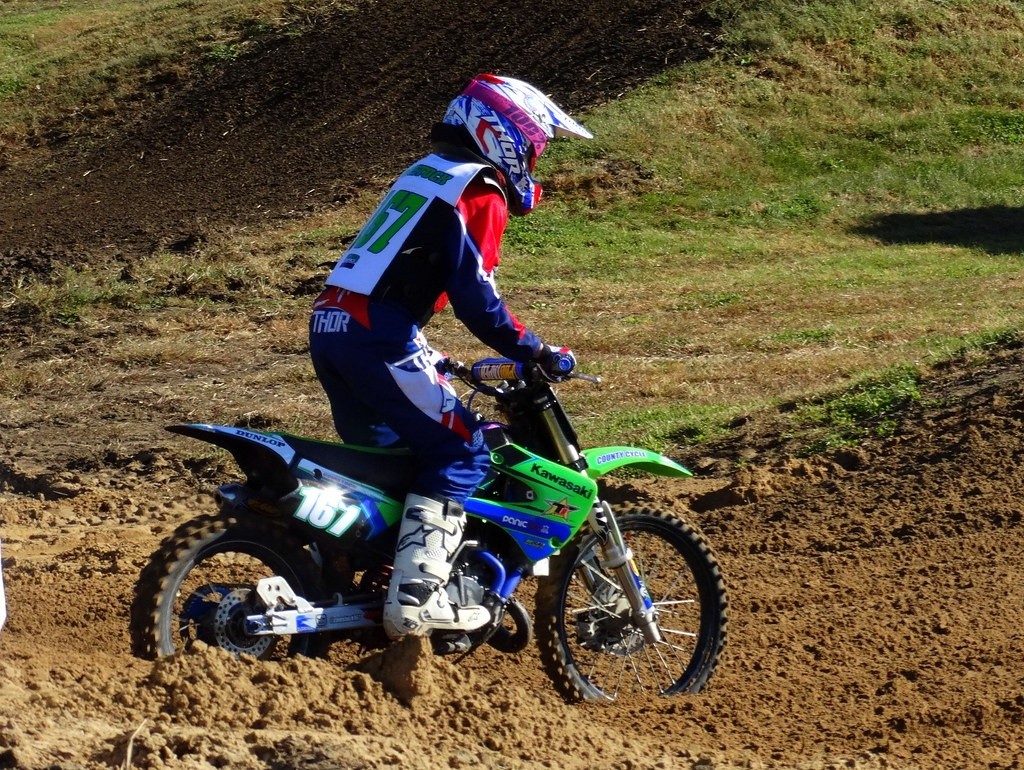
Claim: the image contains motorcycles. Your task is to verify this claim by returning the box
[130,342,729,715]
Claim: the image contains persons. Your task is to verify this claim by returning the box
[307,73,595,642]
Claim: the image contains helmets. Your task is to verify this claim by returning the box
[442,74,594,216]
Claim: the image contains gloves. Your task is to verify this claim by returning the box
[538,345,576,383]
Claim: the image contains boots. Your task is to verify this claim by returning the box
[383,494,491,639]
